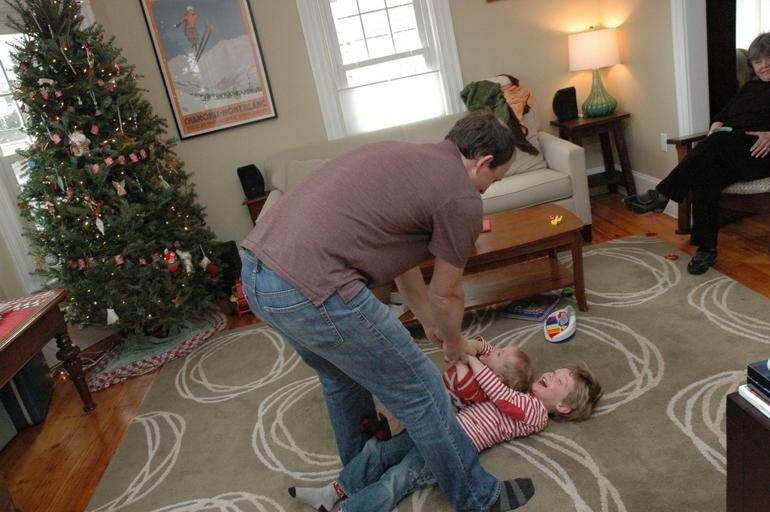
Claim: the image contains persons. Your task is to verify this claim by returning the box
[734,47,755,89]
[441,331,535,407]
[168,4,210,57]
[240,108,536,510]
[287,351,605,510]
[620,32,770,276]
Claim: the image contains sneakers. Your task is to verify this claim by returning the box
[622,190,669,215]
[688,247,717,274]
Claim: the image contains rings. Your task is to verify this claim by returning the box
[764,146,768,151]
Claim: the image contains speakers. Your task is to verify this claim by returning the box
[553,86,579,121]
[236,163,267,200]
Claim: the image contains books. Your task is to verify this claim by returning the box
[498,293,560,321]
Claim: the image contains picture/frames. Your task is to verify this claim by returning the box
[139,1,279,142]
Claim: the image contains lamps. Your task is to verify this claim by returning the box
[567,26,621,118]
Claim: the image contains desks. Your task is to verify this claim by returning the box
[549,111,636,197]
[0,287,98,412]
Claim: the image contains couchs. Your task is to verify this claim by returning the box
[254,101,592,244]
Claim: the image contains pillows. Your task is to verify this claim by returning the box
[503,106,548,177]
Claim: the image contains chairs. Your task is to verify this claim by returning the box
[666,128,770,235]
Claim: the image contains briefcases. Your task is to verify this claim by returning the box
[0,351,53,429]
